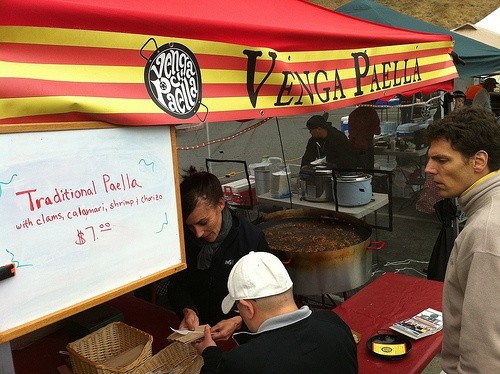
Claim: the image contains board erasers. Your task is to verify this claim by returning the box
[0,258,17,283]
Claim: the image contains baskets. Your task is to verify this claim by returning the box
[131,341,205,374]
[66,321,154,374]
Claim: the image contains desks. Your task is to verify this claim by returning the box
[332,274,444,373]
[257,138,430,219]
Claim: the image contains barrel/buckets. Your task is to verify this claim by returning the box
[254,166,277,195]
[272,171,291,195]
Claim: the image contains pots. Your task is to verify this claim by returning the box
[252,207,385,295]
[331,171,373,205]
[300,171,334,201]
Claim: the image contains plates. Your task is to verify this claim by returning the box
[366,333,412,358]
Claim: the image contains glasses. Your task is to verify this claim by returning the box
[231,299,256,313]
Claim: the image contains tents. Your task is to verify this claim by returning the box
[0,0,500,152]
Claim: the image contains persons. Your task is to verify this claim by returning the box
[473,78,499,112]
[434,90,466,120]
[296,100,381,188]
[191,251,358,374]
[168,171,271,342]
[424,106,500,374]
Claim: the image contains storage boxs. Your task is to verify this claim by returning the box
[220,175,255,206]
[59,321,204,374]
[341,116,429,137]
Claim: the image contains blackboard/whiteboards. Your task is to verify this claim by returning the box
[0,121,187,345]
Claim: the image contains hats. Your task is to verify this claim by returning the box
[307,115,332,130]
[220,251,294,314]
[484,78,499,84]
